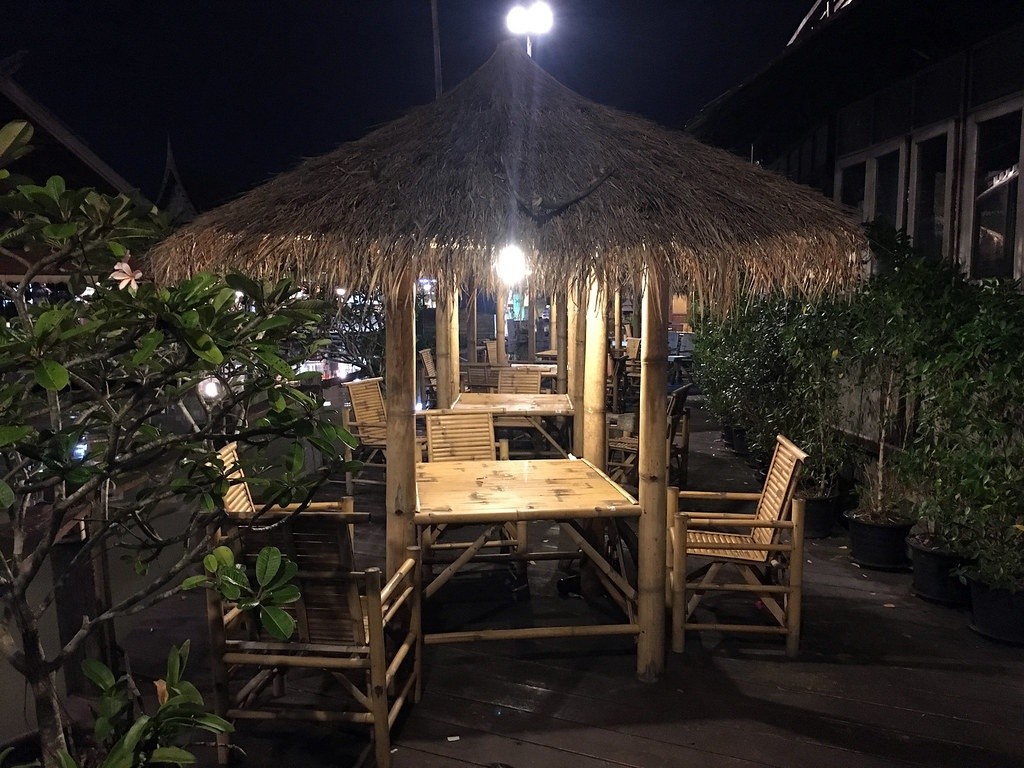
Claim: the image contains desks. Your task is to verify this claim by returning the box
[513,363,560,391]
[535,348,558,362]
[449,393,578,462]
[409,456,646,703]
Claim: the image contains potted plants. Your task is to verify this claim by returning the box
[700,245,1024,643]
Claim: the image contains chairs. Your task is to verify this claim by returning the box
[203,313,814,768]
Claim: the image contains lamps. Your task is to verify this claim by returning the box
[668,288,688,316]
